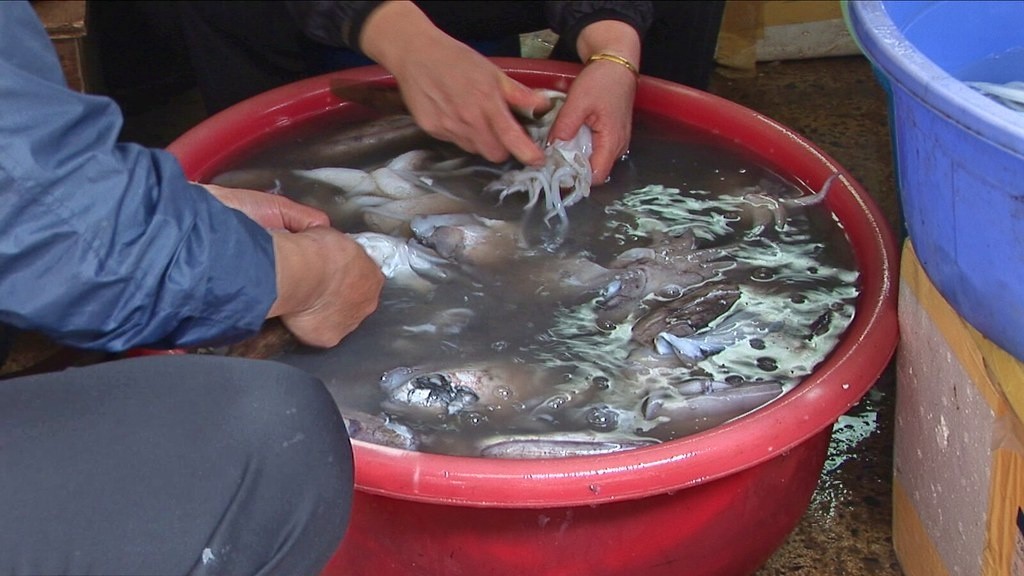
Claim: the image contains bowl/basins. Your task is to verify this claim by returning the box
[840,0,1024,364]
[119,56,899,574]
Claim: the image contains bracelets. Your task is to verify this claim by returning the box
[586,53,641,79]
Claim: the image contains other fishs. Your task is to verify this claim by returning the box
[337,177,842,464]
[292,151,532,293]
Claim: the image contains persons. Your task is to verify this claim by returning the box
[0,0,386,576]
[83,1,654,185]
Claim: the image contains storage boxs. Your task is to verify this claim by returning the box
[712,1,862,66]
[886,235,1024,574]
